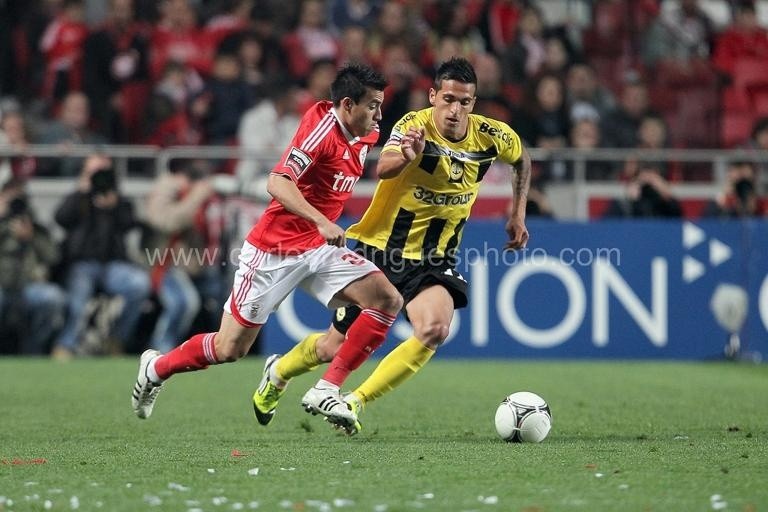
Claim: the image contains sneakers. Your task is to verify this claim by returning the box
[131,349,164,420]
[302,386,362,436]
[253,354,288,426]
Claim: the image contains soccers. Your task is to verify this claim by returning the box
[495,392,551,442]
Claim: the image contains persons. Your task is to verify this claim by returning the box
[1,156,212,362]
[2,1,767,219]
[133,58,532,437]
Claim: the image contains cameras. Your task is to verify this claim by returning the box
[525,200,540,215]
[10,197,25,214]
[91,169,114,191]
[640,184,654,198]
[735,179,753,198]
